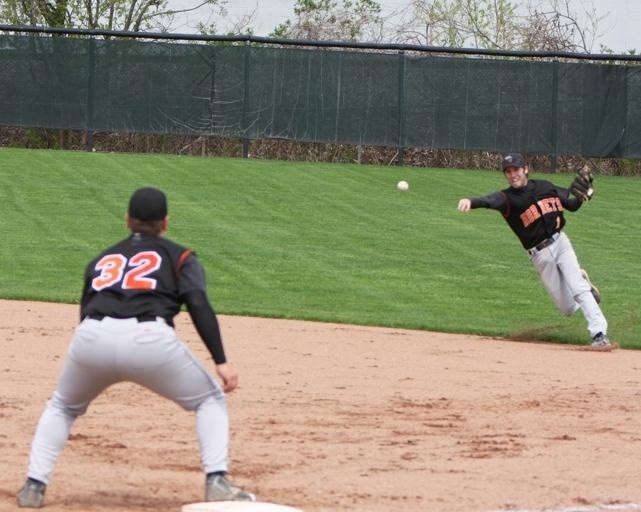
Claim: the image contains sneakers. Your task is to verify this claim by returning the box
[15,479,46,507]
[205,474,256,502]
[581,269,601,304]
[591,335,611,348]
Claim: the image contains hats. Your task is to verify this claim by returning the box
[128,187,167,221]
[501,154,525,172]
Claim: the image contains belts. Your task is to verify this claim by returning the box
[528,238,554,255]
[89,312,155,322]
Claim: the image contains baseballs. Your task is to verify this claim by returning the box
[395,181,410,191]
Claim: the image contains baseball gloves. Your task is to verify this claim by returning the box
[568,172,595,201]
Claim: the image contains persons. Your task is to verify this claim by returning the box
[458,151,614,348]
[14,187,259,510]
